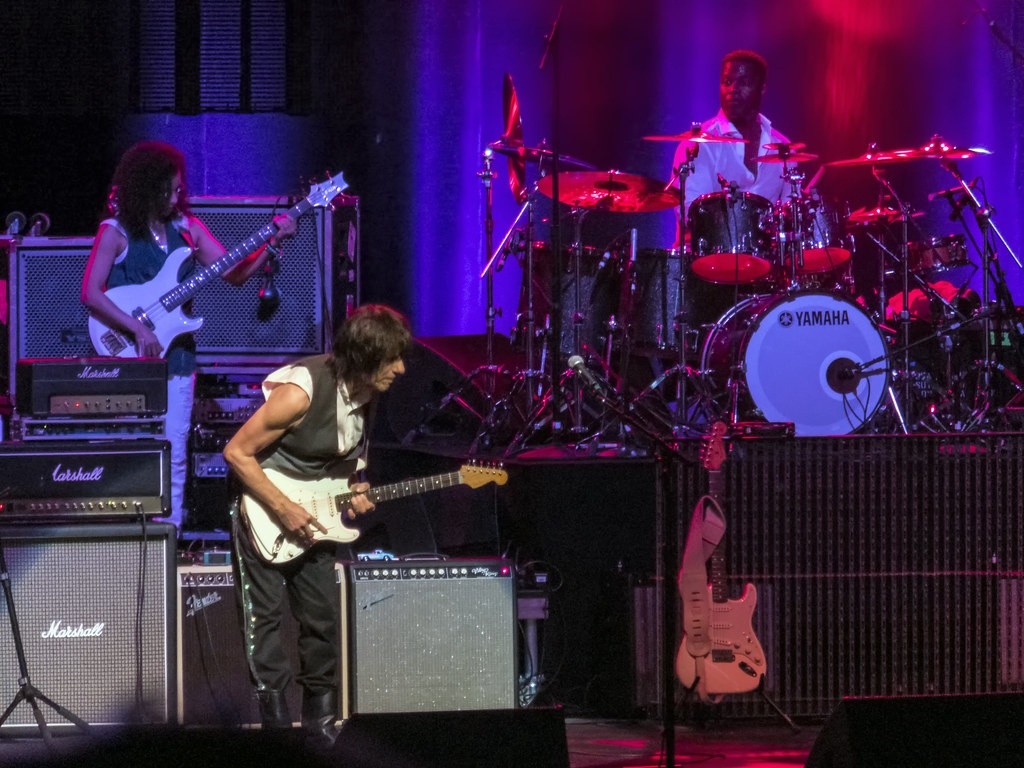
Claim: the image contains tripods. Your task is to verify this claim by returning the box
[403,148,1024,457]
[1,537,96,752]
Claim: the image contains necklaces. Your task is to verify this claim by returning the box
[149,227,167,251]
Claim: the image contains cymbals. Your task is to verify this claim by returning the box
[502,71,528,205]
[826,142,995,168]
[751,141,820,164]
[642,131,751,144]
[537,169,686,214]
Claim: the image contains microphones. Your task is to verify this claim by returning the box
[566,354,603,390]
[259,286,282,303]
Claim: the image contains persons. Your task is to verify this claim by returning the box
[81,140,299,539]
[223,305,409,745]
[670,48,829,253]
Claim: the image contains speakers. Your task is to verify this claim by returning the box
[805,693,1024,768]
[332,556,519,721]
[0,521,177,736]
[328,707,571,768]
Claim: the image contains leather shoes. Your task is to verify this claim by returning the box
[300,692,340,759]
[257,690,293,740]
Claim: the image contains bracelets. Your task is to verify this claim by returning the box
[267,241,279,255]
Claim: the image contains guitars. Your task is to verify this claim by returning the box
[88,169,351,361]
[240,458,511,569]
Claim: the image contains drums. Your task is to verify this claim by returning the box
[507,241,623,360]
[892,232,970,282]
[612,246,719,362]
[700,290,892,435]
[687,189,776,284]
[778,190,856,273]
